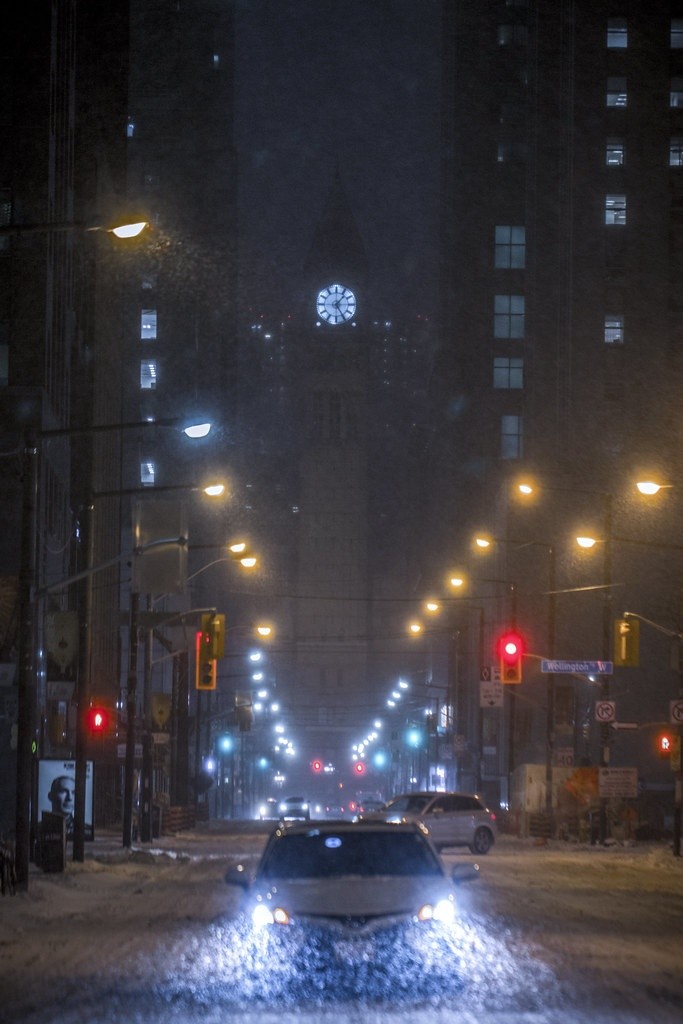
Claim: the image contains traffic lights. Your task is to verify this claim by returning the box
[658,734,671,752]
[88,708,105,733]
[311,755,321,773]
[353,758,365,774]
[196,632,215,689]
[499,636,521,684]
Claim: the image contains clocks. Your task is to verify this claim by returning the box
[315,281,358,326]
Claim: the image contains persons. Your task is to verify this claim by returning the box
[37,776,93,840]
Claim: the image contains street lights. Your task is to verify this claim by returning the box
[11,416,272,895]
[349,679,450,793]
[256,734,287,819]
[405,478,615,842]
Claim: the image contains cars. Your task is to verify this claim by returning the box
[261,798,282,821]
[277,795,311,823]
[316,791,388,821]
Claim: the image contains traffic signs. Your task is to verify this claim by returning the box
[608,718,641,732]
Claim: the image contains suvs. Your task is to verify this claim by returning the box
[358,791,498,854]
[225,818,480,1002]
